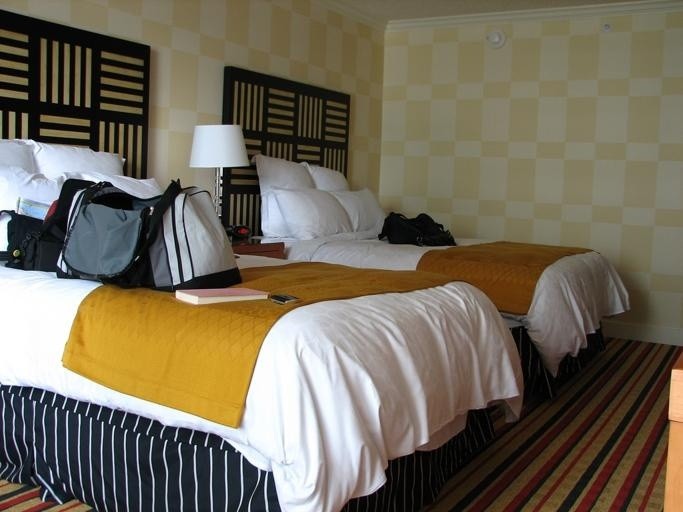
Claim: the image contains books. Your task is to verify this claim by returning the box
[174,285,271,305]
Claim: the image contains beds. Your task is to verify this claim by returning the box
[220,66,632,399]
[0,8,524,511]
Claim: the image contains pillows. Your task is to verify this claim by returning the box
[253,155,386,241]
[0,138,166,211]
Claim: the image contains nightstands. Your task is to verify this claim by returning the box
[230,236,285,260]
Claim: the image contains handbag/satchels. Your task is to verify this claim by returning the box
[382,213,456,246]
[0,178,242,292]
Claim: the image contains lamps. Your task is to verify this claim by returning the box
[189,124,250,224]
